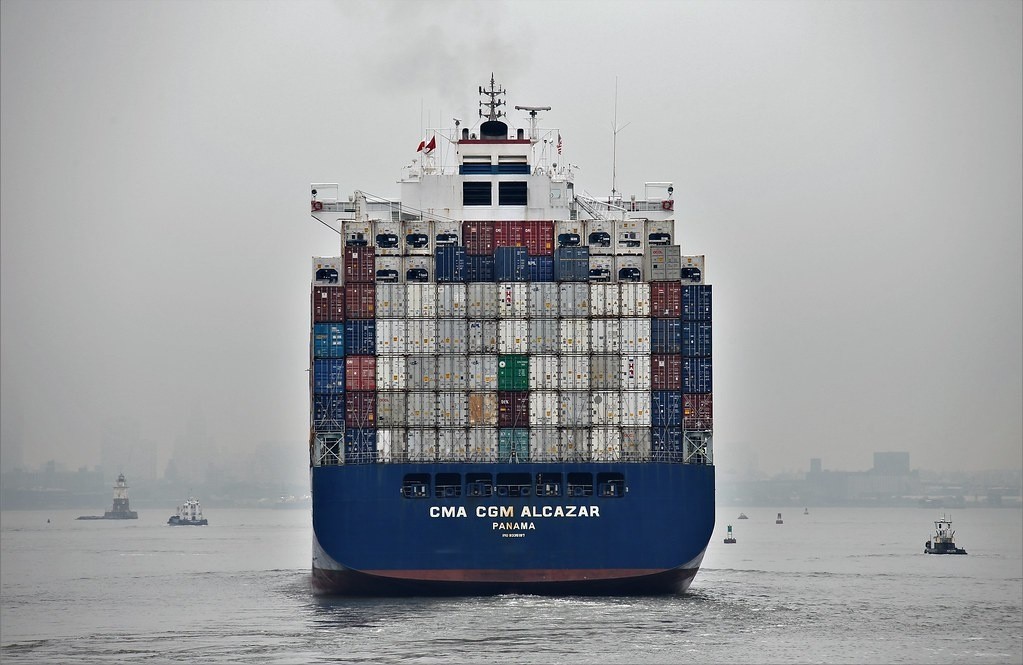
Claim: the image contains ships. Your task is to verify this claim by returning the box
[311,70,716,597]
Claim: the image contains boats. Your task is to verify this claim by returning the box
[723,524,736,543]
[776,512,783,524]
[923,513,967,556]
[166,496,209,527]
[104,473,139,519]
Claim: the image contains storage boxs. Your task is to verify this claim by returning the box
[310,219,713,466]
